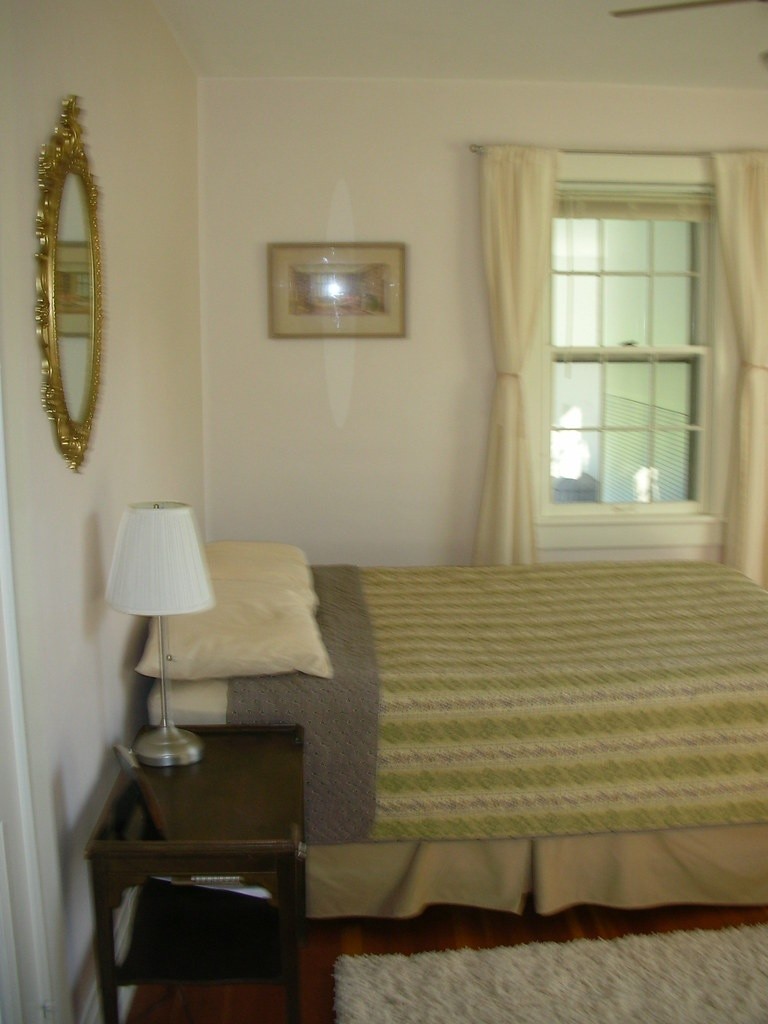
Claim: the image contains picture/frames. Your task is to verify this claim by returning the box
[34,94,103,472]
[46,234,93,341]
[267,242,407,337]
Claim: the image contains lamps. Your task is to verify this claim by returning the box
[104,501,215,766]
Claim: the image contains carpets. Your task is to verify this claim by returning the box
[333,923,768,1023]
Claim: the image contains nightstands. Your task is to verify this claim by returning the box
[84,724,307,1024]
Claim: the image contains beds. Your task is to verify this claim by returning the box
[146,559,768,919]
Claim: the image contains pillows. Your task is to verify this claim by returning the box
[135,541,336,680]
